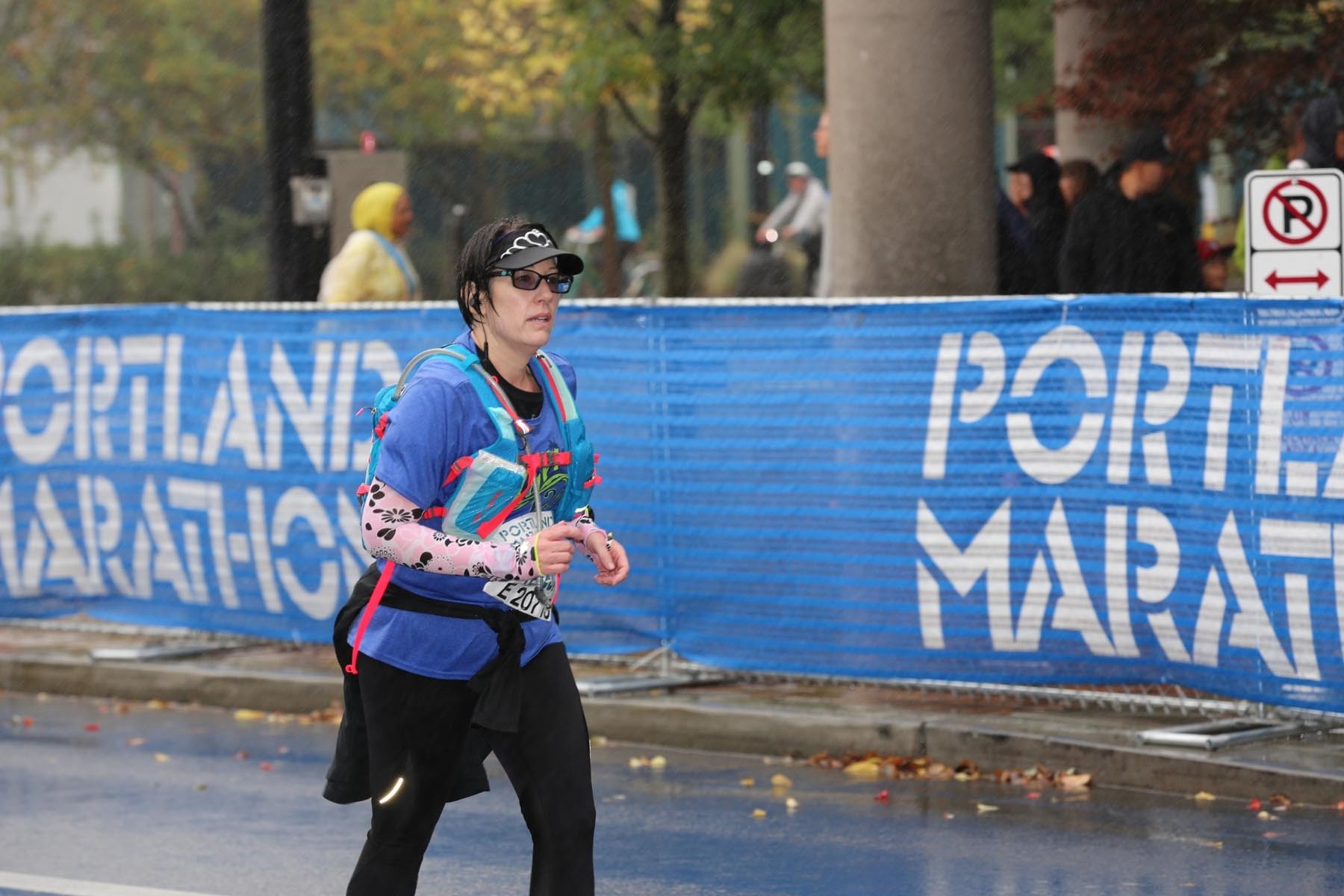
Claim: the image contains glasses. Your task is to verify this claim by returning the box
[486,269,573,293]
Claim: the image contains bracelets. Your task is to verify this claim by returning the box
[534,534,545,576]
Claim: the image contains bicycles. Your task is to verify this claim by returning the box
[562,225,640,298]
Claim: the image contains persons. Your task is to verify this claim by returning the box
[345,217,630,896]
[314,181,424,302]
[562,129,1236,294]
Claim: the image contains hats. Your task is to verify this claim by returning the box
[482,226,585,275]
[1122,126,1181,164]
